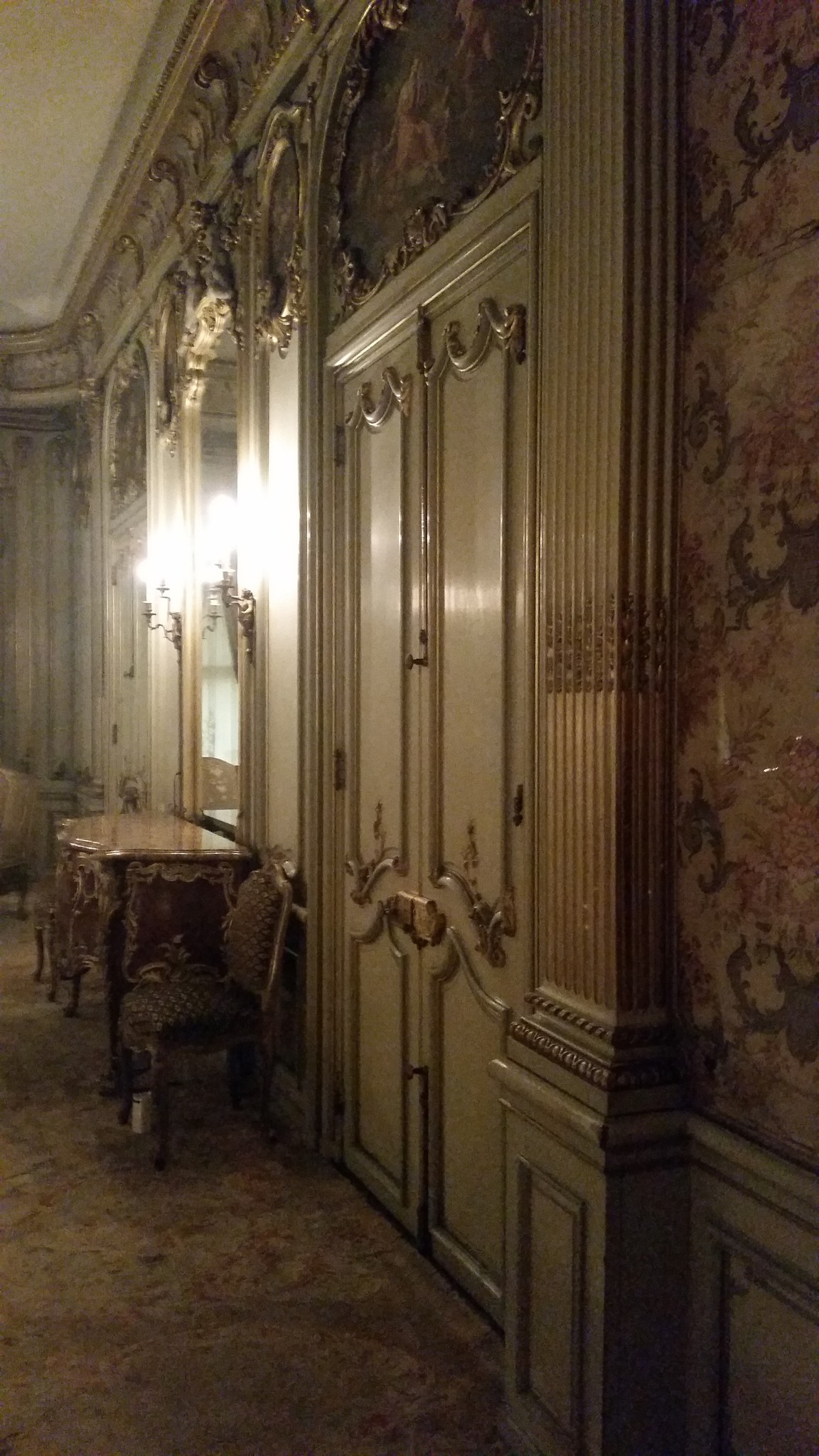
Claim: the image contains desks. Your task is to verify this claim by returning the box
[41,807,260,1063]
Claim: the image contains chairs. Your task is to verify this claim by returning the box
[104,859,294,1170]
[1,768,38,907]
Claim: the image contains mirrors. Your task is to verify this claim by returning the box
[102,368,158,797]
[195,325,243,823]
